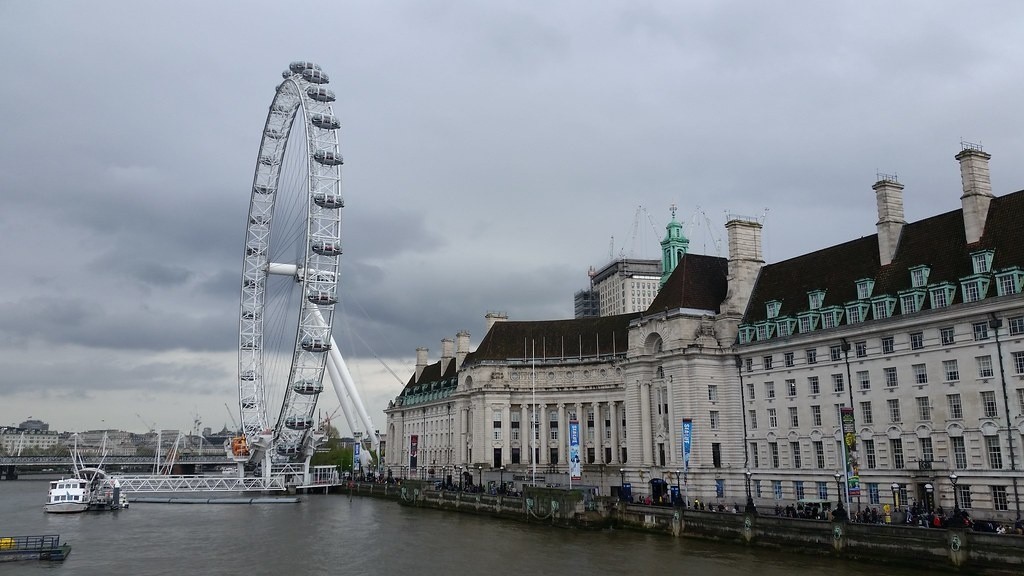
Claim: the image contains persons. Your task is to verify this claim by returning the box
[775,502,831,520]
[639,492,670,505]
[686,497,742,513]
[96,483,99,495]
[507,481,521,498]
[387,465,392,477]
[854,504,946,527]
[108,489,110,498]
[110,477,115,490]
[454,483,497,494]
[435,484,440,491]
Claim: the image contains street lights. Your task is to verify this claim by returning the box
[443,466,446,489]
[833,472,845,522]
[404,464,406,485]
[500,468,504,496]
[388,464,391,484]
[675,469,681,510]
[421,465,425,484]
[948,474,963,529]
[745,470,751,515]
[348,464,351,478]
[479,466,482,494]
[459,466,462,490]
[373,465,376,482]
[619,468,624,505]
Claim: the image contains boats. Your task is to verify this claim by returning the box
[43,478,129,513]
